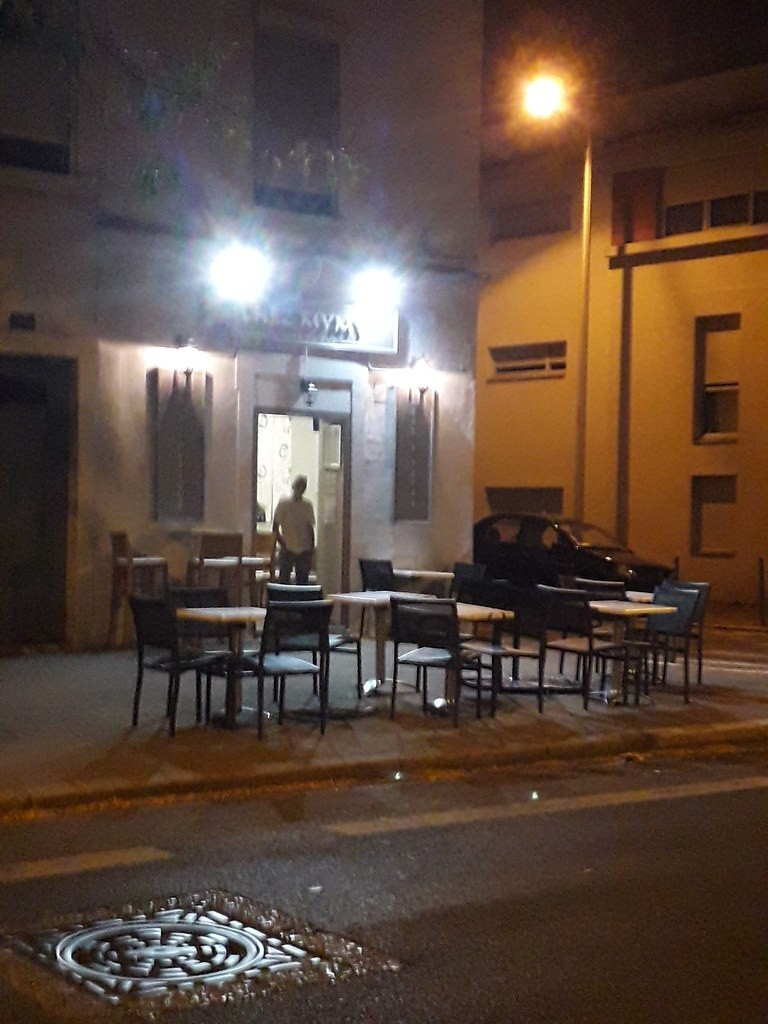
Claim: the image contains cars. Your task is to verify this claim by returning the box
[474,507,675,602]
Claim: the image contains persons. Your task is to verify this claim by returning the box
[272,474,316,585]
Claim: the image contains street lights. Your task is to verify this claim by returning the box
[524,77,598,544]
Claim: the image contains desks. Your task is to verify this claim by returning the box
[399,601,514,719]
[176,607,270,727]
[327,591,438,696]
[393,569,454,598]
[588,600,678,705]
[605,591,666,684]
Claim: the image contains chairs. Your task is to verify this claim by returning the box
[108,531,710,735]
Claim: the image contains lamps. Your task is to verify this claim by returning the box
[302,381,317,408]
